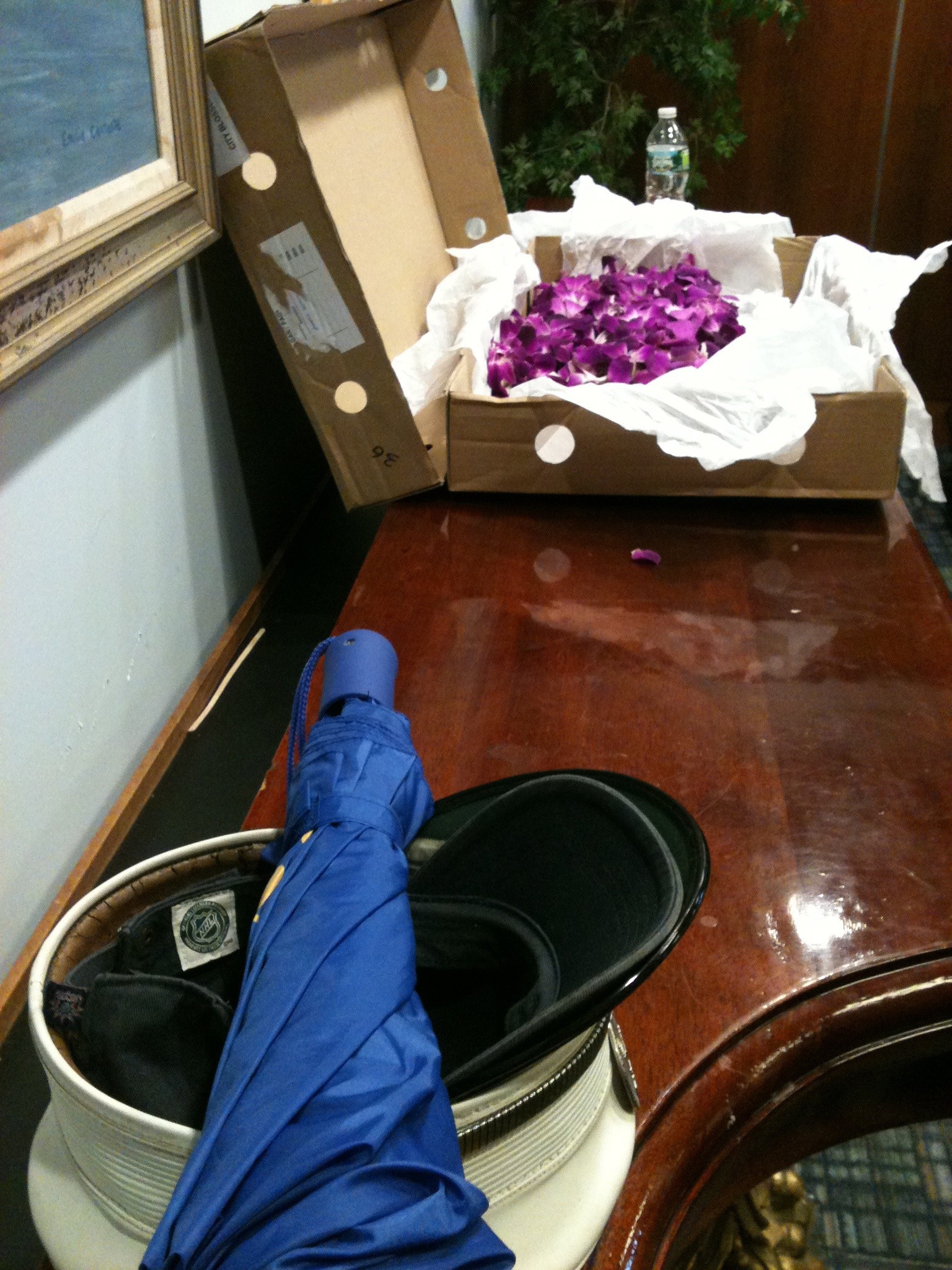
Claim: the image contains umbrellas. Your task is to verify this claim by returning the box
[140,629,518,1269]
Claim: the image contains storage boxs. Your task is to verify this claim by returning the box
[199,0,907,509]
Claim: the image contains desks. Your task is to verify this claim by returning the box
[247,499,951,1270]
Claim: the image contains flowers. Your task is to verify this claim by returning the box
[486,251,745,398]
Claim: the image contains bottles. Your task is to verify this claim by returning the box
[645,106,689,205]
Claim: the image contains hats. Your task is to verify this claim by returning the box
[19,768,710,1270]
